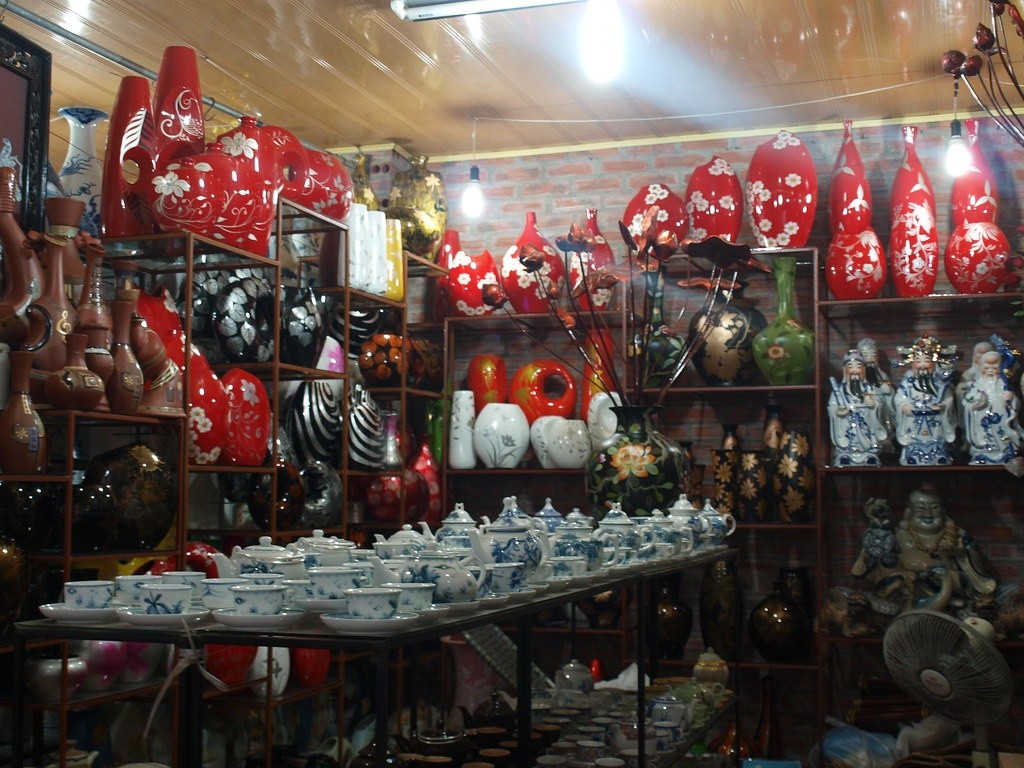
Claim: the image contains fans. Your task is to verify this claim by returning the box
[883,609,1012,768]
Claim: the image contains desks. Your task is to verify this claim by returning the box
[12,545,750,766]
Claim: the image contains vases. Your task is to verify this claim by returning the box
[642,570,691,656]
[1,45,1013,525]
[697,556,743,659]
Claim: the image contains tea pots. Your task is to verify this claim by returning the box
[664,677,725,711]
[286,492,734,571]
[44,739,99,768]
[207,538,293,578]
[554,659,594,690]
[457,692,516,735]
[348,738,407,768]
[499,680,569,723]
[693,647,728,688]
[606,710,654,751]
[389,719,483,762]
[466,497,549,586]
[375,543,487,602]
[634,693,701,727]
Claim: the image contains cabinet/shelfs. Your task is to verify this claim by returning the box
[0,192,1024,768]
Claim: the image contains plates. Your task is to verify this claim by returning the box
[38,603,120,624]
[320,611,419,634]
[212,607,306,627]
[526,583,549,593]
[418,604,451,619]
[442,601,482,614]
[548,573,596,592]
[115,607,210,626]
[482,589,538,609]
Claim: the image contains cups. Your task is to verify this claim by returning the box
[398,677,732,768]
[63,571,436,619]
